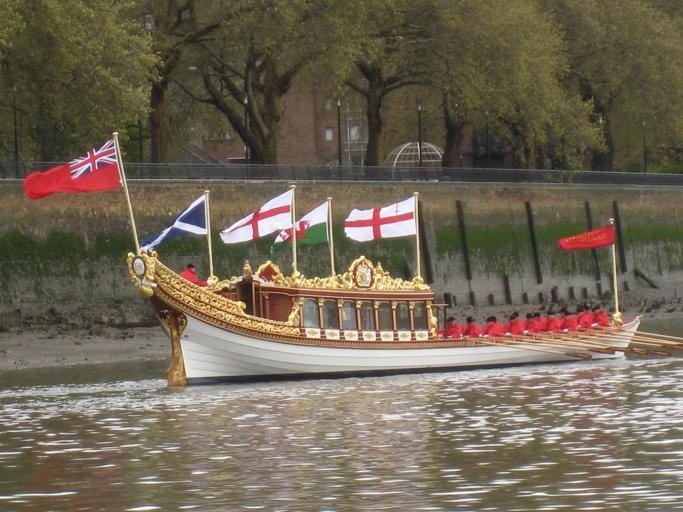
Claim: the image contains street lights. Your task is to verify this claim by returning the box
[642,120,650,173]
[11,85,18,178]
[484,111,489,166]
[416,96,422,167]
[243,94,248,164]
[336,97,342,165]
[597,113,604,171]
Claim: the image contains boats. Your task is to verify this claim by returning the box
[126,250,647,387]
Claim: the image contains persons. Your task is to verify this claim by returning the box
[442,305,611,339]
[179,264,213,287]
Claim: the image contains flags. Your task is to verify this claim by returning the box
[343,195,416,243]
[219,188,293,244]
[274,201,330,244]
[556,224,615,252]
[139,194,208,253]
[22,139,122,200]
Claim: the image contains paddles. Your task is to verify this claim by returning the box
[458,328,682,360]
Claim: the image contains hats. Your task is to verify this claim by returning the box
[446,305,600,322]
[187,264,194,267]
